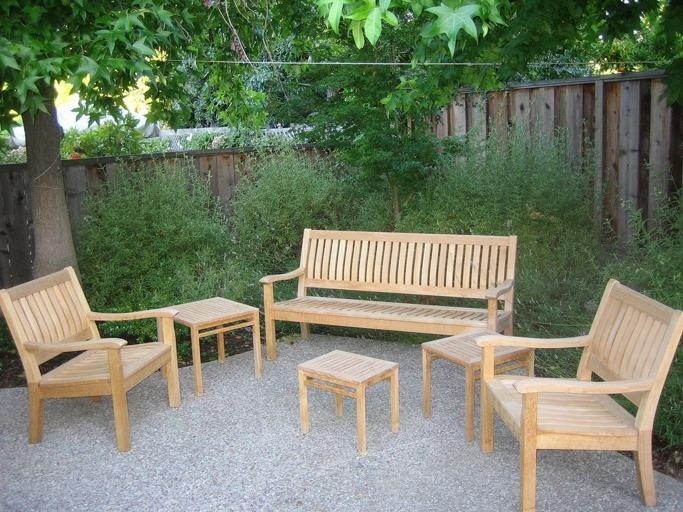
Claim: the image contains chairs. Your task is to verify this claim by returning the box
[474,277,683,512]
[0,265,182,453]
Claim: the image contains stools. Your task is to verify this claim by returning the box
[155,295,264,398]
[296,349,400,452]
[421,329,536,443]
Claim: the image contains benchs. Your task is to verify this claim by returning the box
[258,227,518,363]
